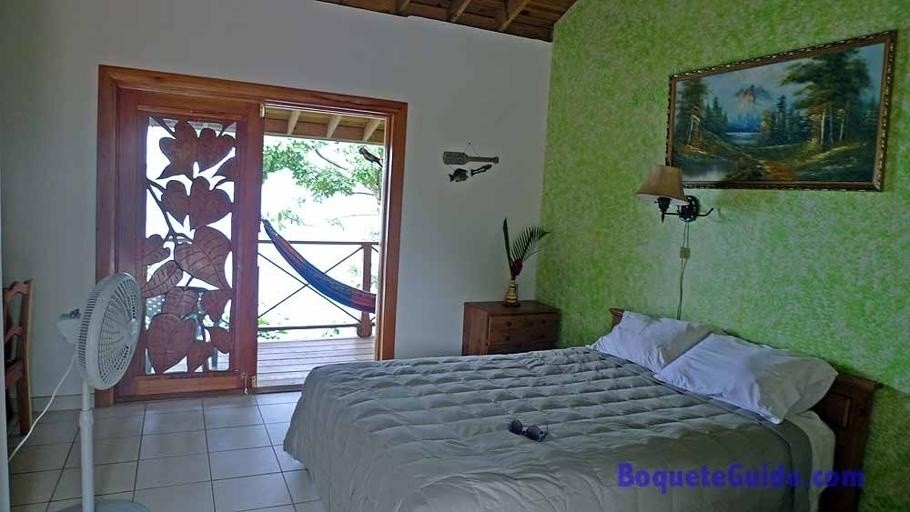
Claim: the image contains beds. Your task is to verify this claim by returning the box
[285,307,885,512]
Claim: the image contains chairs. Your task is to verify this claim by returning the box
[2,279,34,436]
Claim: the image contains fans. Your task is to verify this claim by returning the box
[52,273,151,512]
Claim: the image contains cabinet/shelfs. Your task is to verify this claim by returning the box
[461,300,563,356]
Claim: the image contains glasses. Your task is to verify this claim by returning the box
[508,420,549,442]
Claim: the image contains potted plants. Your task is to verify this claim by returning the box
[502,218,550,307]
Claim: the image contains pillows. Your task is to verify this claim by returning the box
[584,312,840,425]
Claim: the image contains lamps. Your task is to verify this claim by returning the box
[634,163,713,223]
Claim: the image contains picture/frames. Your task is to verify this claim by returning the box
[664,32,896,192]
